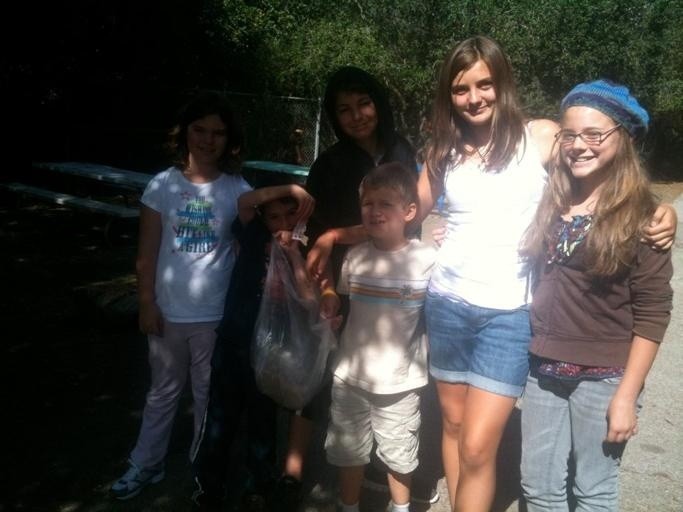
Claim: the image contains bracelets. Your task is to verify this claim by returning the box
[318,289,341,309]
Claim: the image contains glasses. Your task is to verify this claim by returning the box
[555,123,622,144]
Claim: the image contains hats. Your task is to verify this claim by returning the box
[560,78,650,141]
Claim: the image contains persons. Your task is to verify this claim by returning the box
[324,162,438,512]
[274,67,439,512]
[214,176,316,512]
[305,34,678,512]
[108,93,254,512]
[431,78,674,512]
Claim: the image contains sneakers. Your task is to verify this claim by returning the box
[112,464,166,500]
[361,468,439,504]
